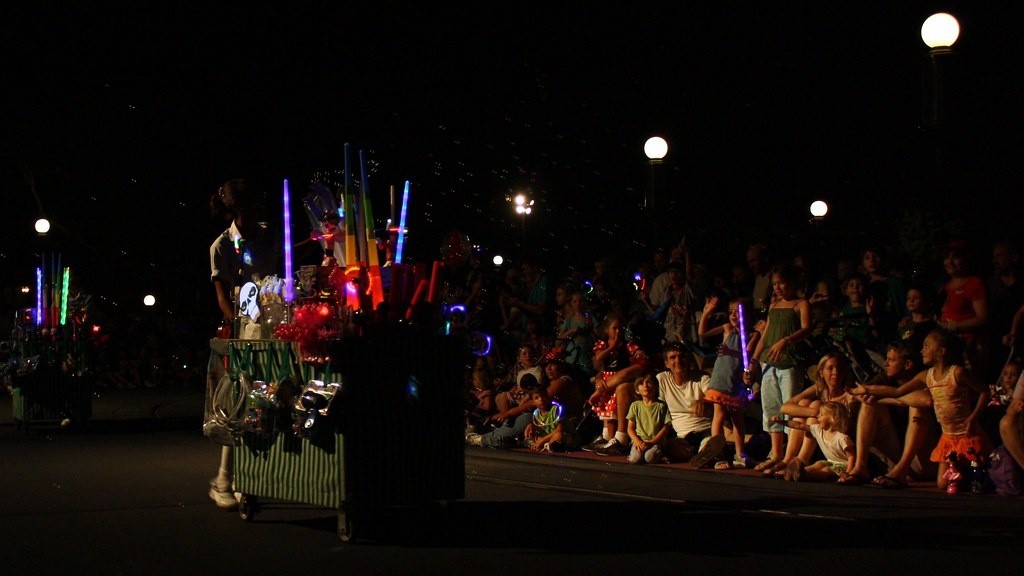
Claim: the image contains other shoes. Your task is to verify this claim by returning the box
[715,460,730,469]
[764,462,785,475]
[784,460,794,482]
[544,441,564,453]
[733,456,745,467]
[793,458,805,482]
[753,460,775,472]
[467,433,484,445]
[209,487,237,508]
[660,455,671,463]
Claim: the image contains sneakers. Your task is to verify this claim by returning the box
[582,436,608,451]
[594,439,630,456]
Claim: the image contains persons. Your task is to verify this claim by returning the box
[440,228,1024,495]
[207,179,279,508]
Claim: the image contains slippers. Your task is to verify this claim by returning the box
[835,473,867,485]
[873,475,905,488]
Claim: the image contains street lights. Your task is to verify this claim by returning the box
[920,10,963,245]
[492,254,503,285]
[810,198,829,272]
[642,134,671,253]
[34,217,50,326]
